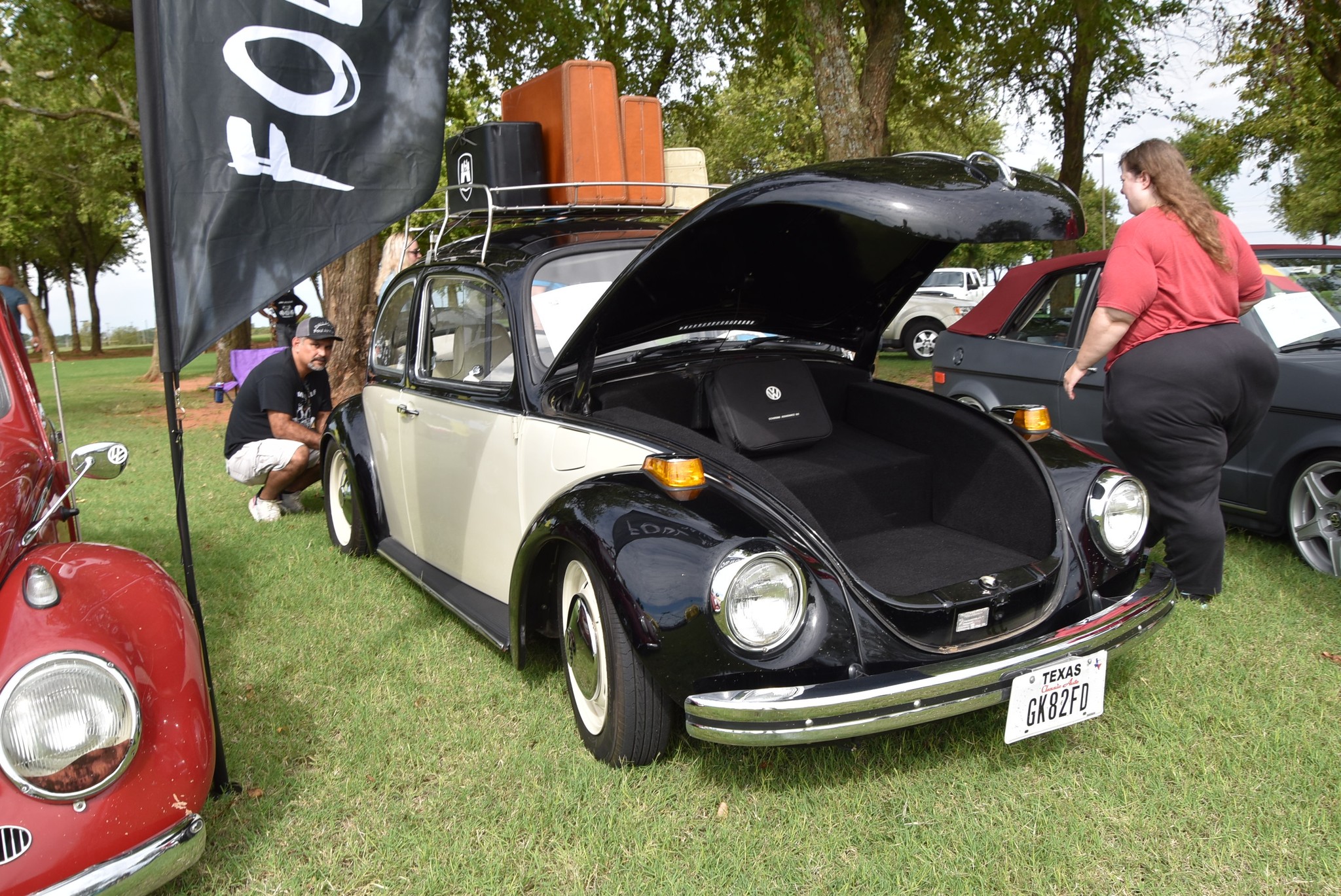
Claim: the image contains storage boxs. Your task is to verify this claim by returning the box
[444,60,710,216]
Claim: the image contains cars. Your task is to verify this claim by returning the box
[914,267,984,302]
[315,149,1182,771]
[931,243,1340,580]
[878,267,989,361]
[0,290,215,896]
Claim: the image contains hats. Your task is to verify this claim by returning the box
[296,317,343,341]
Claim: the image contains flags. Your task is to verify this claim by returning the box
[135,0,451,384]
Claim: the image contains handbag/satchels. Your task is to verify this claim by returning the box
[705,355,834,458]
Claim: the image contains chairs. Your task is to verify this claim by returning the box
[207,347,290,404]
[446,324,514,382]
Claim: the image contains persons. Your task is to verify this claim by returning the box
[257,290,309,348]
[1063,137,1282,606]
[0,266,41,359]
[224,316,345,524]
[373,231,423,308]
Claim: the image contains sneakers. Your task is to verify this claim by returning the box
[248,487,283,524]
[1138,554,1211,610]
[276,491,305,515]
